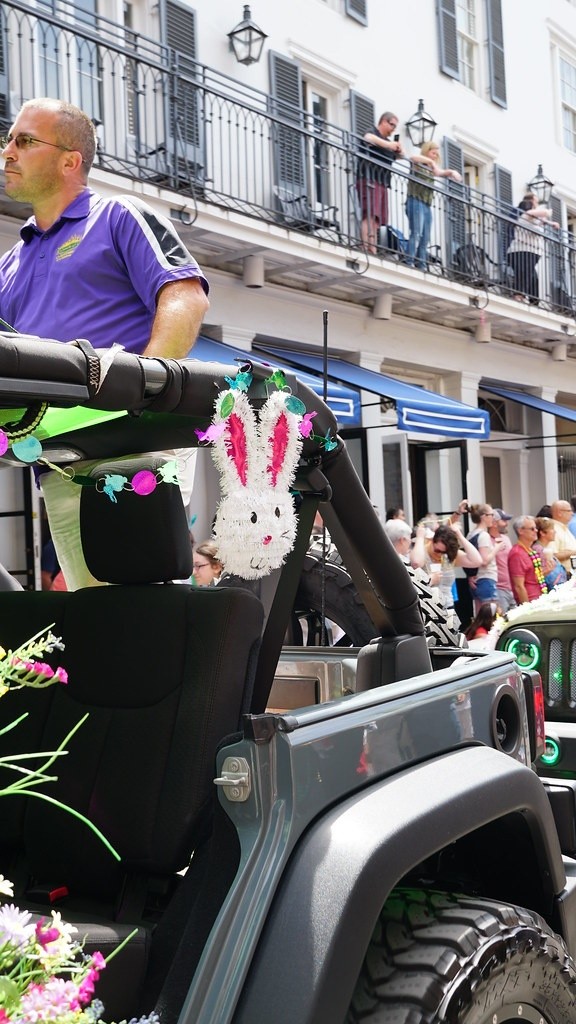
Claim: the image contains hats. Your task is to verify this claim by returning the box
[495,508,512,520]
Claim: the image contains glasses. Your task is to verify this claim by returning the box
[1,132,86,165]
[521,527,538,531]
[484,512,495,518]
[193,560,217,571]
[388,120,396,127]
[434,544,447,554]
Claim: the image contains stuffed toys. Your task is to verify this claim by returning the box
[207,389,303,579]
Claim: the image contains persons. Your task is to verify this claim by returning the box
[404,141,463,271]
[355,111,404,254]
[41,539,60,591]
[400,695,462,756]
[192,540,223,588]
[297,511,346,648]
[0,98,211,592]
[506,193,561,304]
[384,499,576,638]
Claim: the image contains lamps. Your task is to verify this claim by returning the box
[404,98,438,148]
[226,4,270,66]
[527,164,554,204]
[242,255,265,288]
[552,343,567,361]
[372,293,393,320]
[475,322,492,343]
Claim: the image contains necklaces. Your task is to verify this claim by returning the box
[518,541,548,594]
[421,517,452,574]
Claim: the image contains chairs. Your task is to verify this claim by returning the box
[456,244,515,299]
[386,226,444,277]
[0,458,267,1024]
[137,143,214,200]
[556,287,576,320]
[272,184,342,245]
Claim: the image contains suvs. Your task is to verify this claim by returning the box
[480,580,576,858]
[0,335,575,1024]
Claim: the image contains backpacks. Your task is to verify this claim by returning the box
[460,531,479,576]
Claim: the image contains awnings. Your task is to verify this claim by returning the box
[252,343,491,440]
[186,336,359,424]
[479,385,576,449]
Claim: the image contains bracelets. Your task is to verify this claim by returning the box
[455,511,461,515]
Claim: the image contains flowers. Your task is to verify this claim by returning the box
[0,622,123,860]
[0,873,160,1024]
